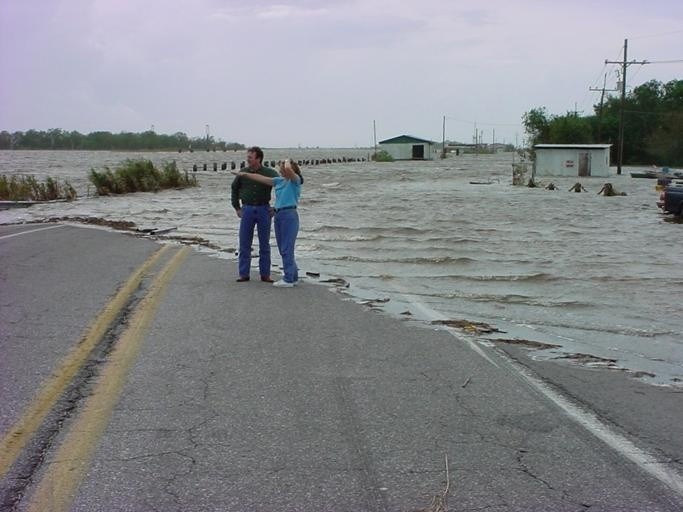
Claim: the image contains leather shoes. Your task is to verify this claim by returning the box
[260,274,276,282]
[236,275,250,282]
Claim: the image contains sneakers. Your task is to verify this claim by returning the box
[271,278,299,289]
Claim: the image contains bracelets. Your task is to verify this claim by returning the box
[284,159,289,162]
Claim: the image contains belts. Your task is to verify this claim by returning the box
[241,199,268,206]
[274,206,297,212]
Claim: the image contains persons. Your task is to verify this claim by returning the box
[229,146,280,283]
[229,157,303,288]
